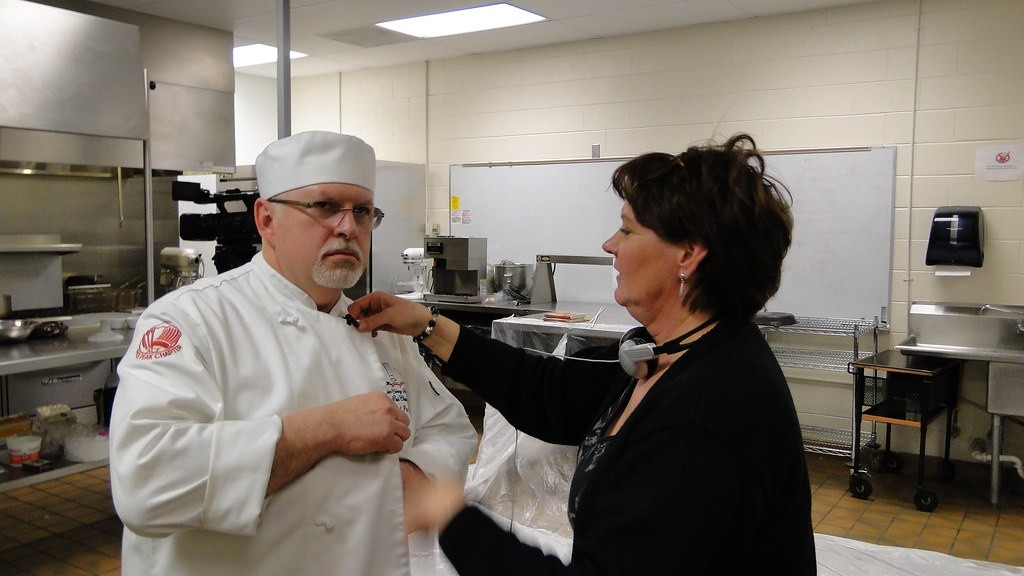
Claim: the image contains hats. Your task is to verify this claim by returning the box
[254,130,376,202]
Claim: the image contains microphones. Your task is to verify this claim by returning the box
[343,314,360,327]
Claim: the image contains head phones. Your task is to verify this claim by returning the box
[617,318,721,379]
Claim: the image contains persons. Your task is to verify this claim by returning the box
[109,131,477,576]
[347,133,817,576]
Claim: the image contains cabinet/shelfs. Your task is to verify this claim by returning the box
[847,350,963,512]
[0,312,146,492]
[758,315,888,477]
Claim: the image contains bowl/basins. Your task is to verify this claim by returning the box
[127,317,139,328]
[0,320,39,339]
[6,436,42,467]
[110,318,126,329]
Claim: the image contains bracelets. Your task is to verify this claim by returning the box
[413,305,439,342]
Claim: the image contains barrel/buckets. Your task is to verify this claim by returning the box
[0,294,11,318]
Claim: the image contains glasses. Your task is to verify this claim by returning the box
[266,198,385,232]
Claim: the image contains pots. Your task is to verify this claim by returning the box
[755,312,800,328]
[495,263,527,293]
[69,289,120,312]
[117,289,141,312]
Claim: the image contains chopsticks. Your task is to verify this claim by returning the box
[586,306,606,327]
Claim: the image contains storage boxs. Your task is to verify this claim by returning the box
[64,289,122,316]
[117,289,143,309]
